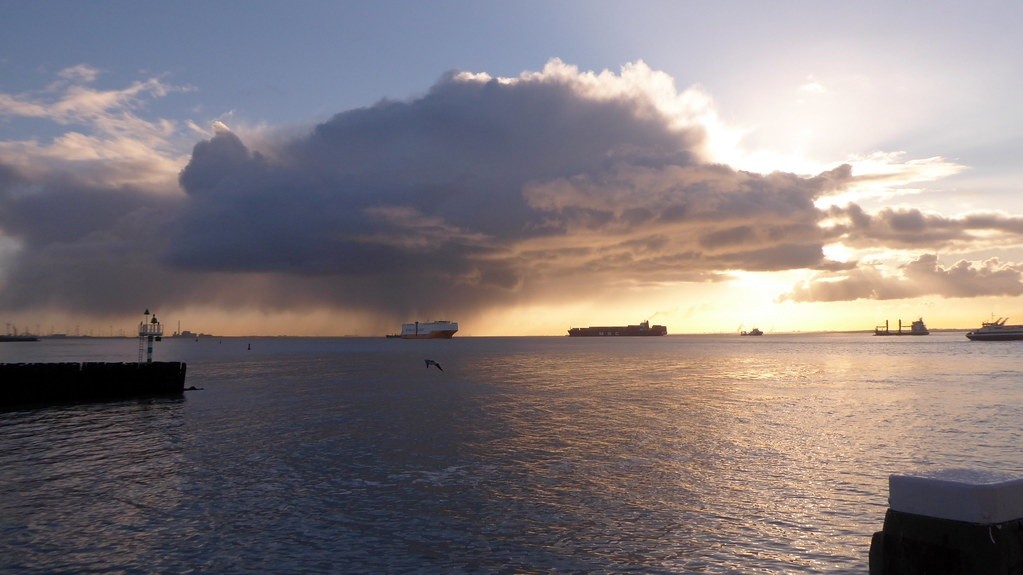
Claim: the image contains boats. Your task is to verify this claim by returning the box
[740,329,763,336]
[873,318,929,336]
[965,317,1023,341]
[385,334,401,338]
[0,334,39,342]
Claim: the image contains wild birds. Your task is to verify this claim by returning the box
[424,359,443,371]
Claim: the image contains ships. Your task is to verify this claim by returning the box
[401,321,458,340]
[568,320,668,336]
